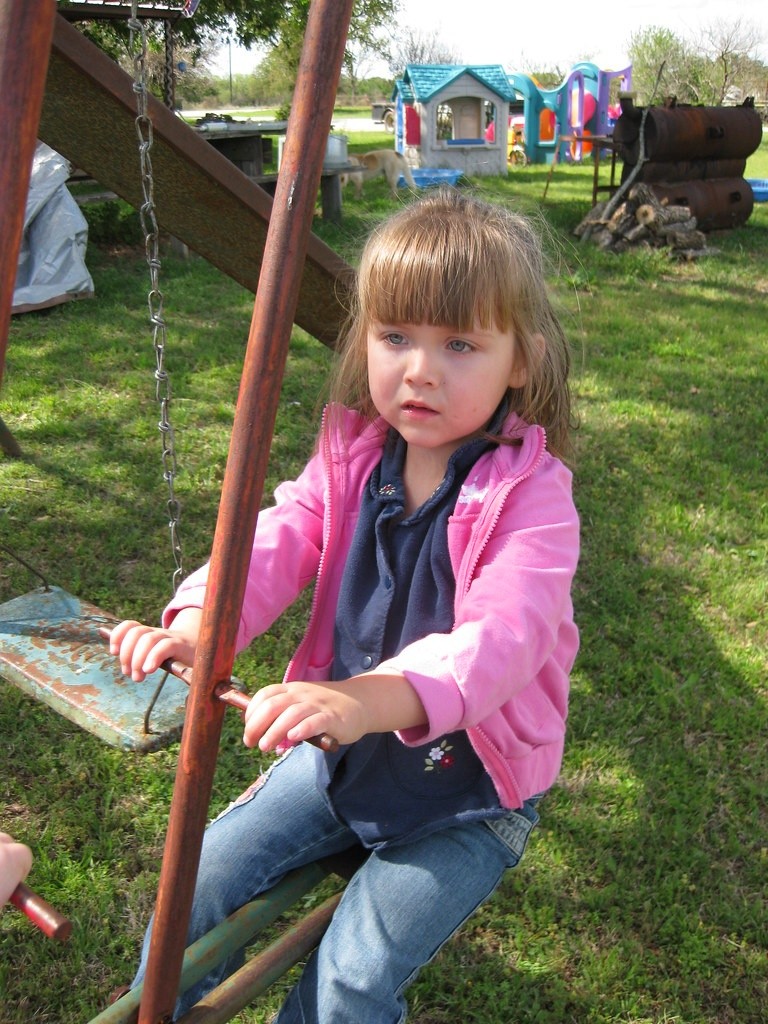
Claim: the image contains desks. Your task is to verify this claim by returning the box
[194,122,290,176]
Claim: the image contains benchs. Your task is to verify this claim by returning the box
[250,162,370,221]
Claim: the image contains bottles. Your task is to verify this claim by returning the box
[200,122,228,132]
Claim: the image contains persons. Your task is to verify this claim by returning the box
[109,185,583,1024]
[0,833,33,916]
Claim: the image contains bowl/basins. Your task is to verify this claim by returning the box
[398,168,464,188]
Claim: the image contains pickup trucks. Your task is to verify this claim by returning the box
[369,101,451,135]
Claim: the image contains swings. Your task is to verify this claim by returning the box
[0,2,190,759]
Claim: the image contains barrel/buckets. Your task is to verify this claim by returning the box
[324,135,349,164]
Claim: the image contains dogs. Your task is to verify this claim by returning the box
[340,148,419,201]
